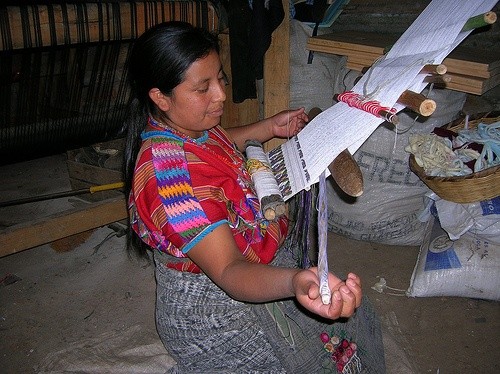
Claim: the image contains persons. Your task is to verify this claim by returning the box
[123,20,388,374]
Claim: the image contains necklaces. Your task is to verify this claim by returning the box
[148,110,247,182]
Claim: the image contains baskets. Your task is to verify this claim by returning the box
[408,116,500,203]
